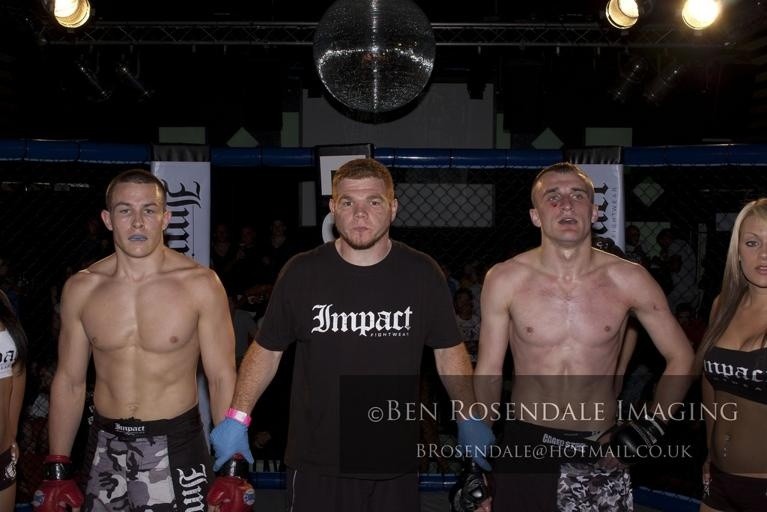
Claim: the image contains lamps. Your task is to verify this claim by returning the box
[39,0,93,34]
[676,1,727,32]
[594,1,651,37]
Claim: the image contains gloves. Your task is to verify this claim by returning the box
[449,473,494,512]
[210,408,256,472]
[208,455,257,512]
[456,416,495,472]
[599,413,667,470]
[33,456,84,512]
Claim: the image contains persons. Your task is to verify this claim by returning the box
[692,197,766,511]
[33,169,258,511]
[0,215,767,509]
[207,159,498,511]
[446,162,697,512]
